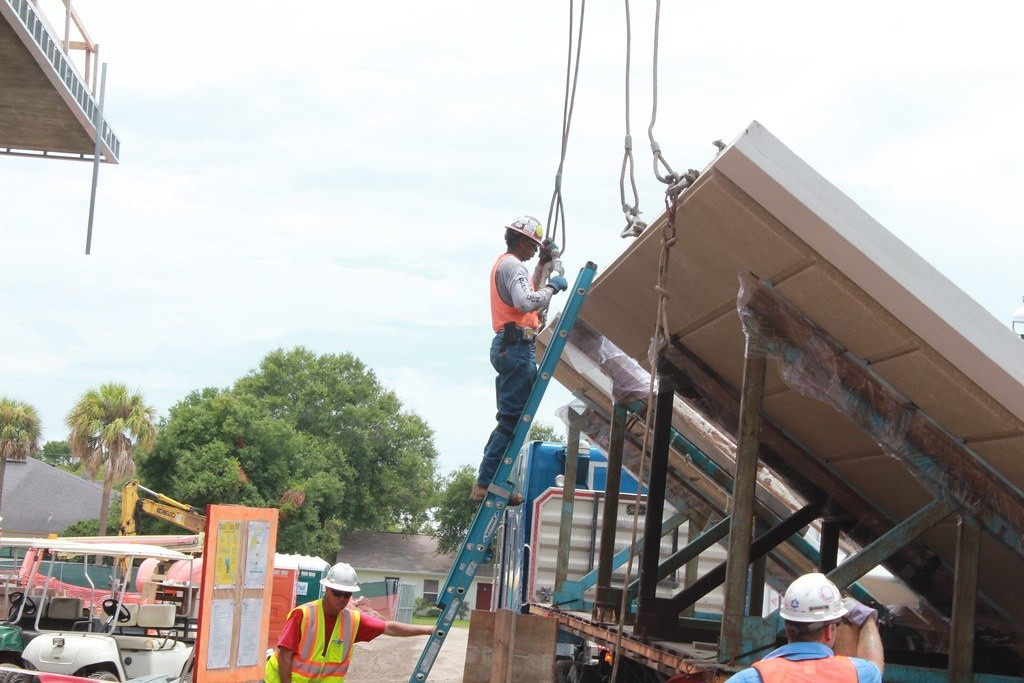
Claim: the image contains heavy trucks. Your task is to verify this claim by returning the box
[500,438,1024,683]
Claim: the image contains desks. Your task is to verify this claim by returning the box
[140,581,200,618]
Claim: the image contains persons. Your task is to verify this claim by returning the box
[724,573,884,683]
[262,562,436,683]
[469,215,568,505]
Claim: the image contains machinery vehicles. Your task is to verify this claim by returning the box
[107,478,206,589]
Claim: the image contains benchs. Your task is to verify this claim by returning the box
[71,604,178,650]
[12,596,93,632]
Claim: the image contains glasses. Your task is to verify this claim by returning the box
[328,587,352,598]
[522,237,538,253]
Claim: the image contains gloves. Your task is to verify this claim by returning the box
[842,598,878,627]
[538,236,556,261]
[545,276,568,295]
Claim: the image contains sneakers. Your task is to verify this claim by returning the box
[471,482,524,505]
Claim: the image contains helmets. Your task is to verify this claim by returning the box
[777,573,847,623]
[506,217,545,247]
[319,562,360,592]
